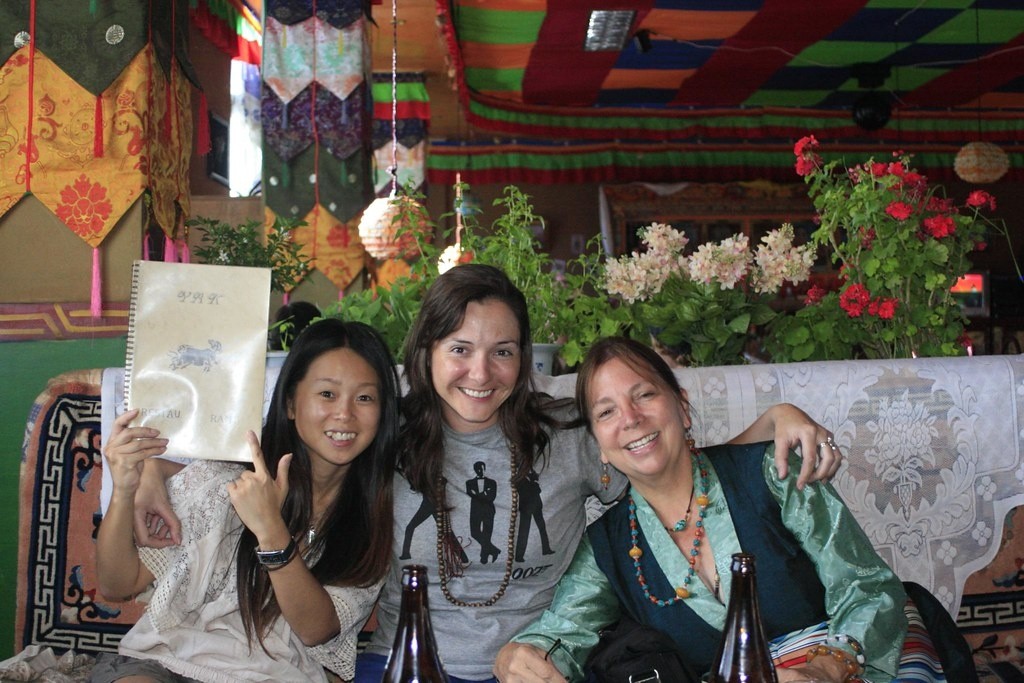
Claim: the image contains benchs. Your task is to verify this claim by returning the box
[0,353,1024,683]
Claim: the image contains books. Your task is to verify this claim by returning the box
[124,258,272,463]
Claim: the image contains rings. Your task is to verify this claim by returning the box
[817,437,837,451]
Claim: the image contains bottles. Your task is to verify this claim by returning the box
[709,553,778,683]
[382,563,447,683]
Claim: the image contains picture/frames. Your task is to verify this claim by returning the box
[514,218,549,253]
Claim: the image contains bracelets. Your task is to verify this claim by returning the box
[263,546,298,571]
[824,634,866,681]
[807,646,856,674]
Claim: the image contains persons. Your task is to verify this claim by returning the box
[94,264,909,683]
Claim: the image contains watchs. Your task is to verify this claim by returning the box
[255,535,294,564]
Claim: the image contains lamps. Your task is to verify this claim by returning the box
[853,103,891,132]
[631,30,652,54]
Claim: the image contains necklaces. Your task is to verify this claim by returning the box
[664,486,694,532]
[627,447,709,607]
[436,426,519,607]
[307,520,316,545]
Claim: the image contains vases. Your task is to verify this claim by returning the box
[533,341,563,377]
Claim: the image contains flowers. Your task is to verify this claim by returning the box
[184,134,998,376]
